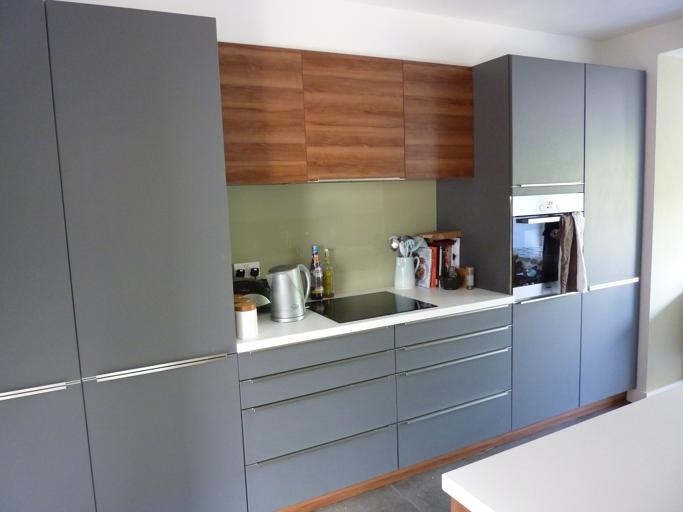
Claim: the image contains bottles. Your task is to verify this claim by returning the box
[466,267,474,289]
[312,245,334,298]
[235,297,258,341]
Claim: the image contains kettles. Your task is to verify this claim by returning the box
[264,263,312,323]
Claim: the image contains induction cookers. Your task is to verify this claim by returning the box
[306,291,442,324]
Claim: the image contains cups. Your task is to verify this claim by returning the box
[394,256,419,289]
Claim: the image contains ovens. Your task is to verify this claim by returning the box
[509,192,584,301]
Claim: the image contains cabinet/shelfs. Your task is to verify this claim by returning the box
[0,2,238,399]
[510,58,586,195]
[301,50,403,181]
[0,376,248,507]
[396,303,514,467]
[513,279,640,430]
[239,324,399,509]
[220,46,304,188]
[585,63,639,287]
[403,60,475,178]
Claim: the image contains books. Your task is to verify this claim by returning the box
[407,228,465,289]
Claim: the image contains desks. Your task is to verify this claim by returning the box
[441,382,682,512]
